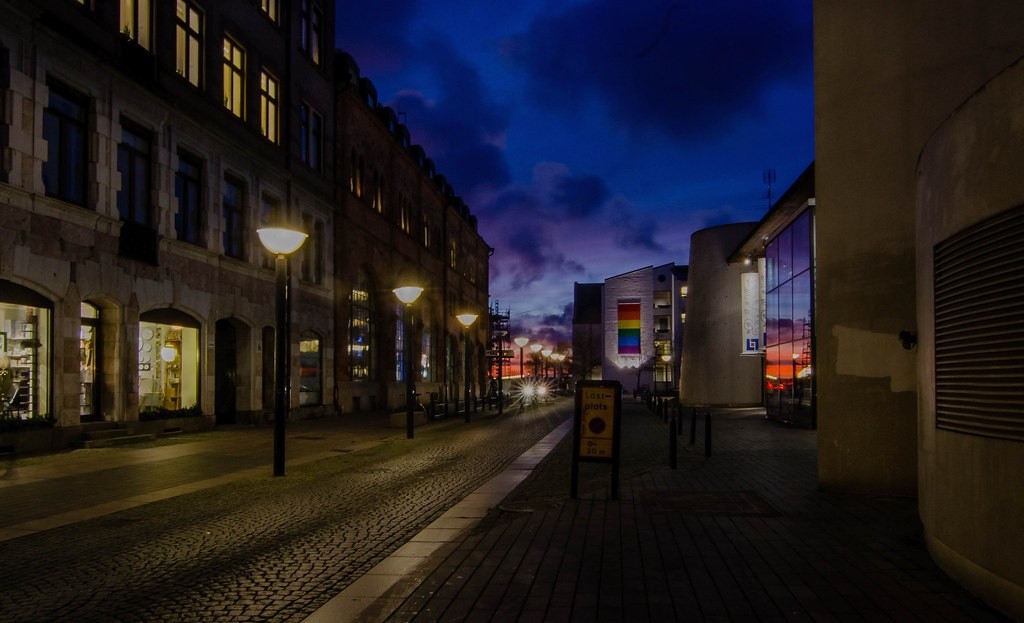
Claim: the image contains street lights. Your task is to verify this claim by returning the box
[392,286,424,439]
[494,331,508,414]
[456,314,479,424]
[256,228,308,478]
[530,344,542,381]
[514,338,530,410]
[551,353,566,398]
[541,349,552,380]
[662,355,671,392]
